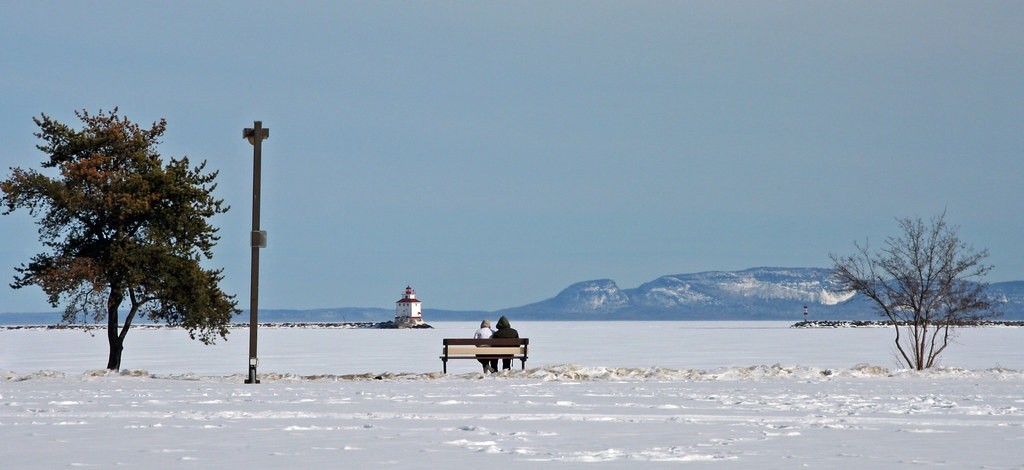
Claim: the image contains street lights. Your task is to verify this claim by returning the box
[244,121,269,384]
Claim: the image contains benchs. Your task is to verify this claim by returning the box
[439,338,529,374]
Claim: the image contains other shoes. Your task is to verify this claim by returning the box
[484,365,494,373]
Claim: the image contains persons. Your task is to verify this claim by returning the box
[473,316,521,374]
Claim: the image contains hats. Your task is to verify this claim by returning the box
[481,320,490,328]
